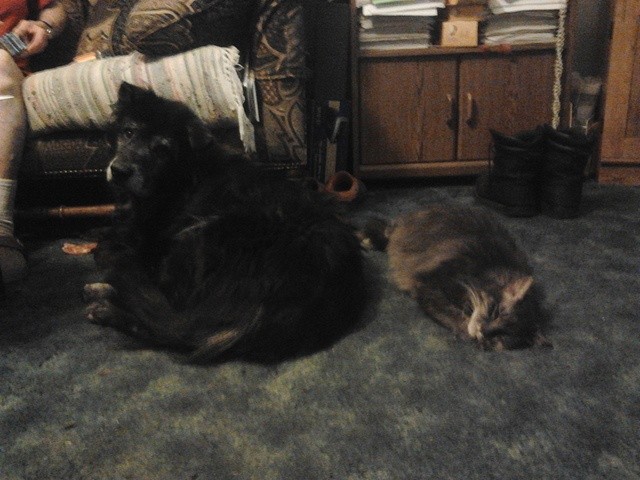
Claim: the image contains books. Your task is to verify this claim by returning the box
[358,3,446,54]
[480,0,563,47]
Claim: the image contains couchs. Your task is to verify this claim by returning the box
[0,0,326,222]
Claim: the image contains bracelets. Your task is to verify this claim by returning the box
[38,15,58,47]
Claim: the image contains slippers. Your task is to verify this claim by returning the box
[0,236,28,283]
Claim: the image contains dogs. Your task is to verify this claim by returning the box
[83,81,368,367]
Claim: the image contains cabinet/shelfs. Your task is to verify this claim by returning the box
[351,0,577,178]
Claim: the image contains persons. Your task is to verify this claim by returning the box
[0,1,86,325]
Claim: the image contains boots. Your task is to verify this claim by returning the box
[544,124,591,221]
[472,125,548,218]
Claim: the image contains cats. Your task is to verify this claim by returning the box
[353,188,555,352]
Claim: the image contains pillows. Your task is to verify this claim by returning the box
[71,0,126,62]
[121,0,254,59]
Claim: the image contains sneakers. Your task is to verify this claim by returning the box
[326,170,369,206]
